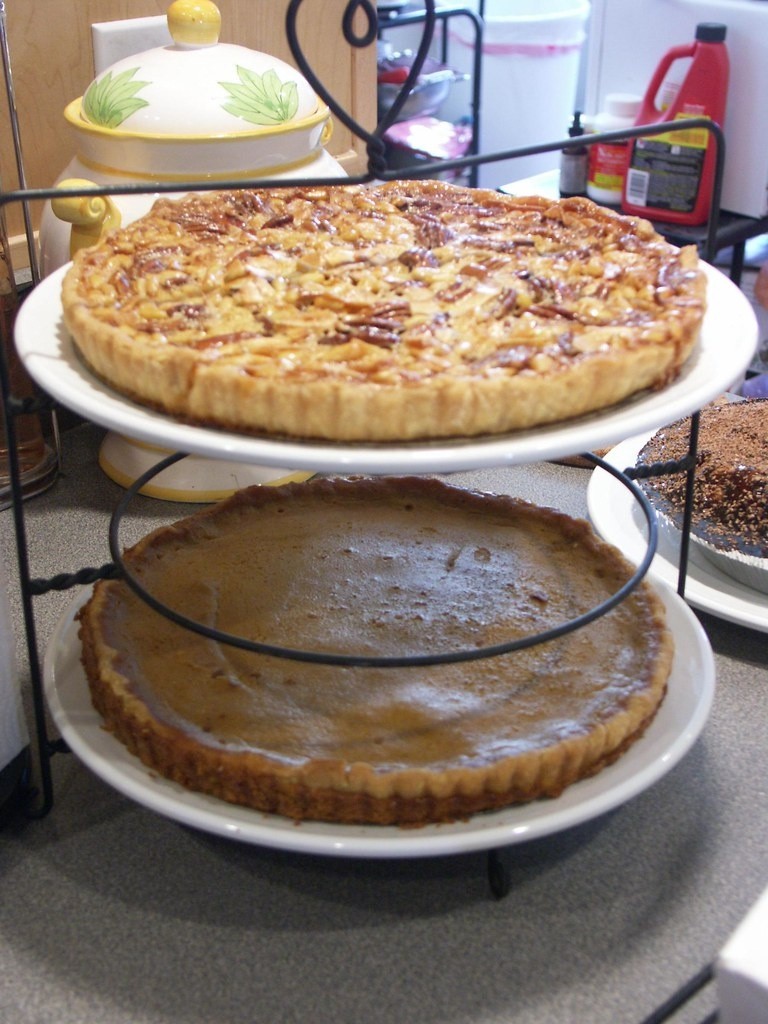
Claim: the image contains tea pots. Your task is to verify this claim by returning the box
[39,2,356,501]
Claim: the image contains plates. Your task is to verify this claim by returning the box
[587,426,768,634]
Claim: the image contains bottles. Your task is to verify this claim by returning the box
[557,110,586,201]
[620,20,730,227]
[586,93,642,204]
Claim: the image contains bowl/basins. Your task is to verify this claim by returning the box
[633,424,768,597]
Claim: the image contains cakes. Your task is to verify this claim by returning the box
[634,397,768,596]
[75,473,674,824]
[60,178,708,440]
